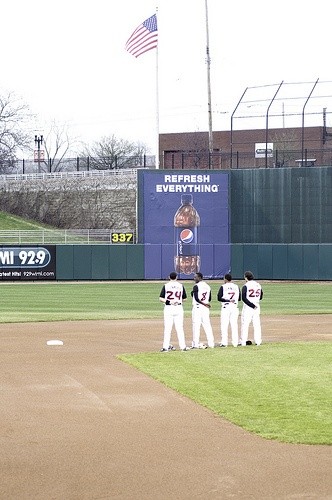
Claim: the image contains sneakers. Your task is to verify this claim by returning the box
[160,348,168,352]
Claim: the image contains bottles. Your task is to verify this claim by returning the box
[174,194,200,275]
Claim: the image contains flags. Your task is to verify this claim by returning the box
[124,13,158,58]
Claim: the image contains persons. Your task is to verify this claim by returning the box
[159,272,190,352]
[238,271,263,346]
[190,273,214,349]
[217,274,241,347]
